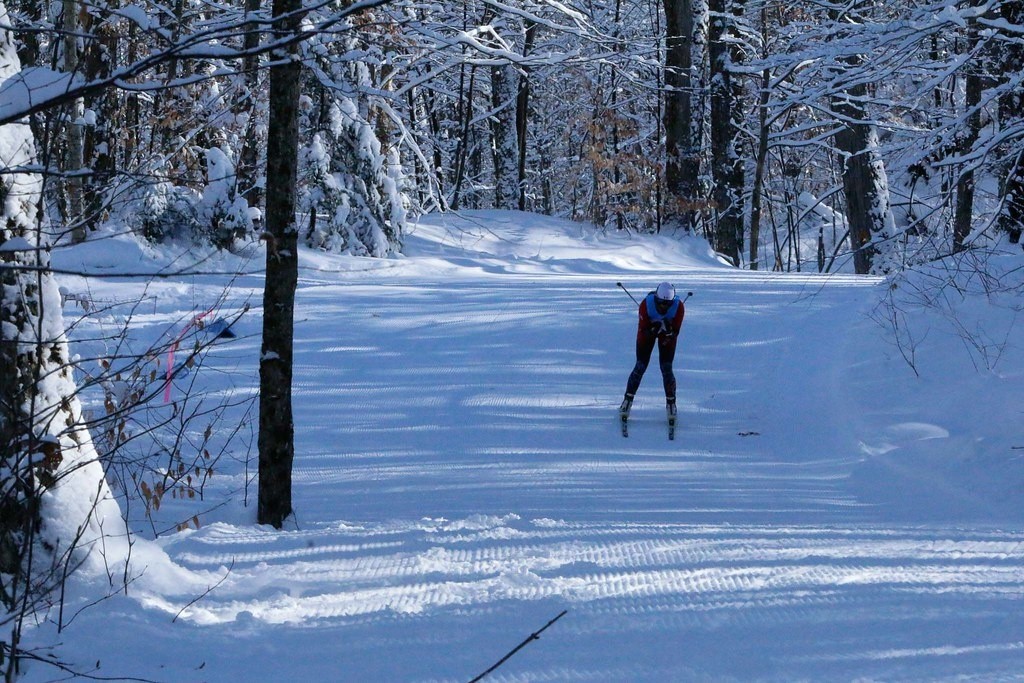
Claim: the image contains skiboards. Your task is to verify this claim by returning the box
[619,407,677,440]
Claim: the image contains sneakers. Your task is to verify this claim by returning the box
[619,399,632,416]
[666,403,677,420]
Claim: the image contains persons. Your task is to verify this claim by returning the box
[618,281,685,420]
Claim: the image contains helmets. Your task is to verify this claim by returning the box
[656,282,675,300]
